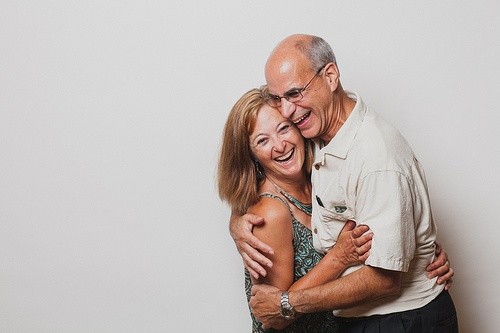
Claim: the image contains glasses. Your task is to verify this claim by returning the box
[262,65,326,108]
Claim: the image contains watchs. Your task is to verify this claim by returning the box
[280,292,296,321]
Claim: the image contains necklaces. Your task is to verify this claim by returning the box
[266,173,312,216]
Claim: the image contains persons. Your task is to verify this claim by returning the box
[216,86,454,333]
[230,34,459,332]
